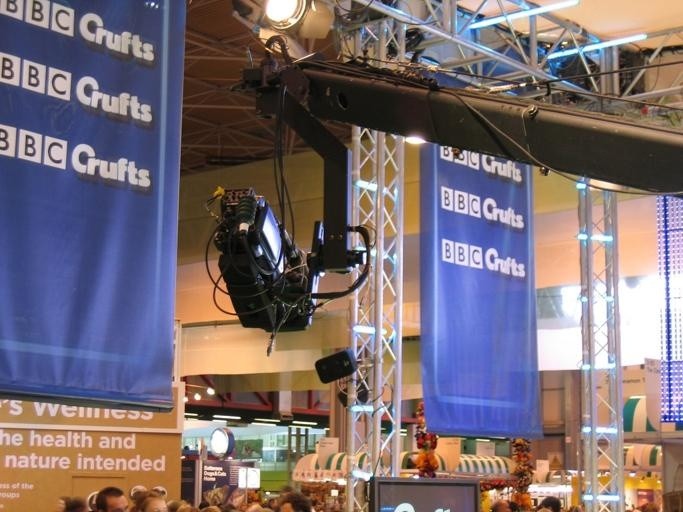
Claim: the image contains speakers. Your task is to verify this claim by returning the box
[336,378,369,407]
[314,348,360,385]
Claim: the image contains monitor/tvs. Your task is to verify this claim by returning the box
[369,476,481,512]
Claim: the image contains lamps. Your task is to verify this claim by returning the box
[263,0,335,40]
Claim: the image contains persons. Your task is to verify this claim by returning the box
[55,479,313,512]
[485,490,583,512]
[640,503,664,512]
